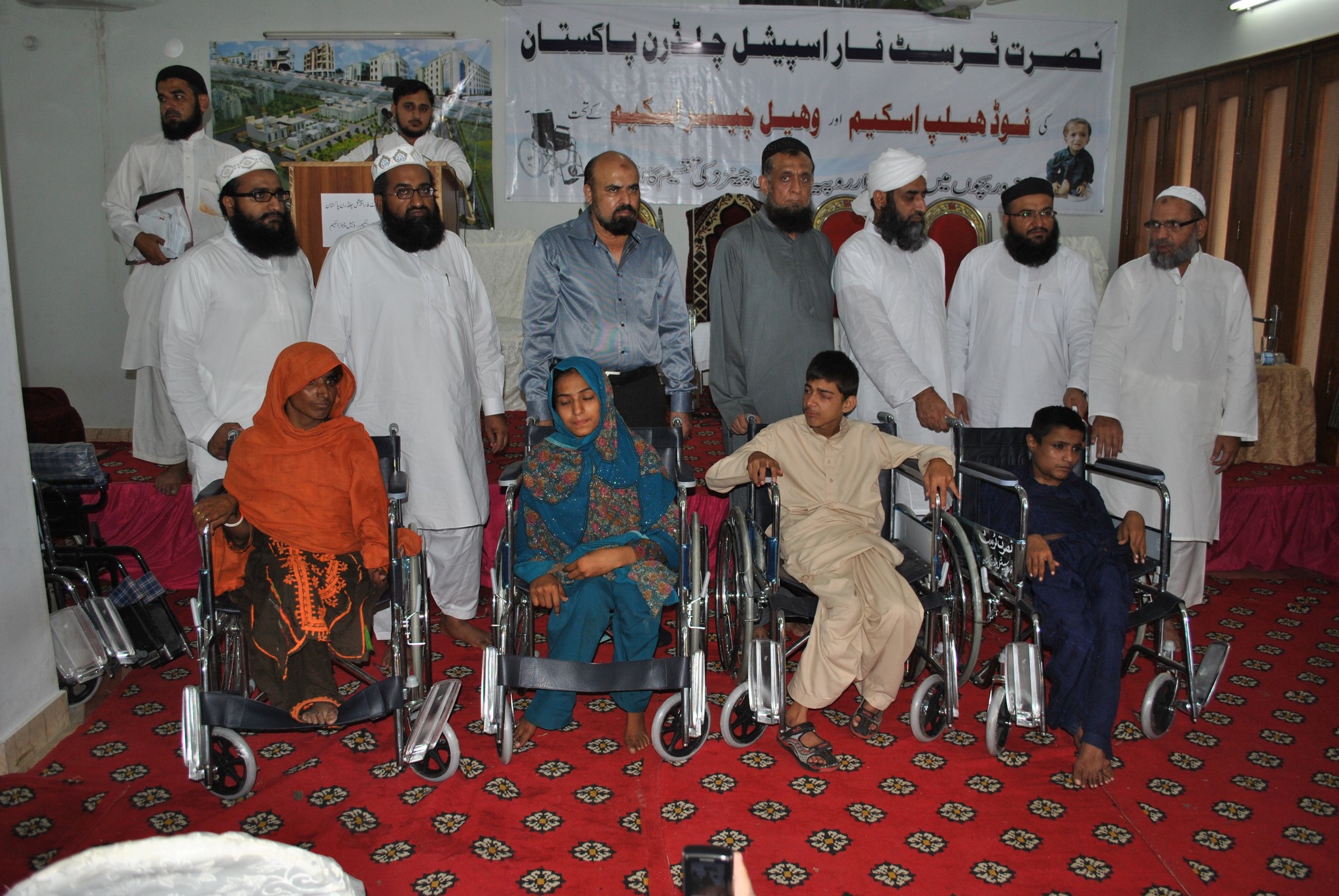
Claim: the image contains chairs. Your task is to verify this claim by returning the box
[812,194,866,348]
[686,193,764,418]
[921,197,992,308]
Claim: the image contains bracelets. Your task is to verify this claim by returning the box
[223,508,244,527]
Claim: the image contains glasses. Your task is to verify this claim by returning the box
[1007,211,1057,221]
[234,190,290,202]
[1143,219,1200,232]
[384,187,437,199]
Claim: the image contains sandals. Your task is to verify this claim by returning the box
[777,720,840,772]
[850,696,882,739]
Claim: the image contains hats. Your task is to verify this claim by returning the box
[1001,177,1054,204]
[867,147,927,199]
[215,149,277,191]
[1155,186,1207,217]
[371,144,429,182]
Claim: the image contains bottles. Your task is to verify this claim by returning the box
[1254,353,1284,366]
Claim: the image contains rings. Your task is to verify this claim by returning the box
[202,516,210,523]
[196,510,200,515]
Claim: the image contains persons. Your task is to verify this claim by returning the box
[307,144,508,648]
[102,64,241,497]
[517,151,696,442]
[157,150,315,505]
[1046,118,1095,200]
[192,342,423,726]
[1088,186,1259,651]
[706,352,957,772]
[514,357,689,754]
[830,148,956,521]
[945,177,1098,428]
[986,406,1146,789]
[708,137,836,515]
[332,78,473,188]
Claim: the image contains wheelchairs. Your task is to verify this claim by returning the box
[715,412,959,748]
[922,418,1231,757]
[517,108,584,186]
[181,423,462,801]
[22,386,197,708]
[480,415,711,765]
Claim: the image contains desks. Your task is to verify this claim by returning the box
[1233,356,1317,465]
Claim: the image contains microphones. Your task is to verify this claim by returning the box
[372,108,432,161]
